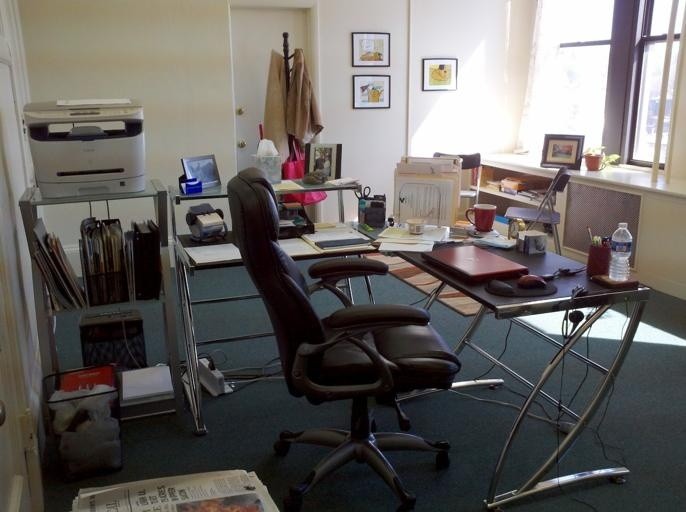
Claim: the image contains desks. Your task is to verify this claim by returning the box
[167,179,363,240]
[177,226,373,436]
[351,225,651,511]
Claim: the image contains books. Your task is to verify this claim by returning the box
[30,217,86,313]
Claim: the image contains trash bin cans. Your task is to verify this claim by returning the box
[37,361,125,487]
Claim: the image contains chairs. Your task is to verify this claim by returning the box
[434,153,571,256]
[227,167,462,511]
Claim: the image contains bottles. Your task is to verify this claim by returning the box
[608,222,633,281]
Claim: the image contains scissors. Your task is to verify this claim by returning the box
[353,184,373,200]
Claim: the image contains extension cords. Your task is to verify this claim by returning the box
[198,357,225,395]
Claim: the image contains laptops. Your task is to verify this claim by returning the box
[420,245,530,286]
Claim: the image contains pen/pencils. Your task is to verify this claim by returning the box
[586,225,612,248]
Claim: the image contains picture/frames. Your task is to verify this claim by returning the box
[540,134,585,170]
[180,155,221,189]
[304,143,342,179]
[352,32,390,108]
[423,58,457,91]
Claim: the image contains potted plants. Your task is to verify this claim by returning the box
[585,145,620,171]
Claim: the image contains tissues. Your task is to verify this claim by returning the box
[250,139,283,185]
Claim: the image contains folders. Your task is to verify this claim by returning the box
[131,217,161,302]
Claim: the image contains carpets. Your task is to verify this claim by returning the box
[364,220,494,317]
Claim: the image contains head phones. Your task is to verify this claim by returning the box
[561,284,588,338]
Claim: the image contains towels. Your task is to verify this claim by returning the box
[262,49,324,164]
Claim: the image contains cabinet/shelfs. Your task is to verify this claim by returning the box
[466,154,562,253]
[18,177,177,436]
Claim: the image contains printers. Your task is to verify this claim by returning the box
[23,96,147,199]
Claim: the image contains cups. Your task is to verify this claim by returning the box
[406,219,427,235]
[586,153,602,171]
[587,244,611,278]
[466,204,497,232]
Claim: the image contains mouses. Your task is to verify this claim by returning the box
[516,274,548,288]
